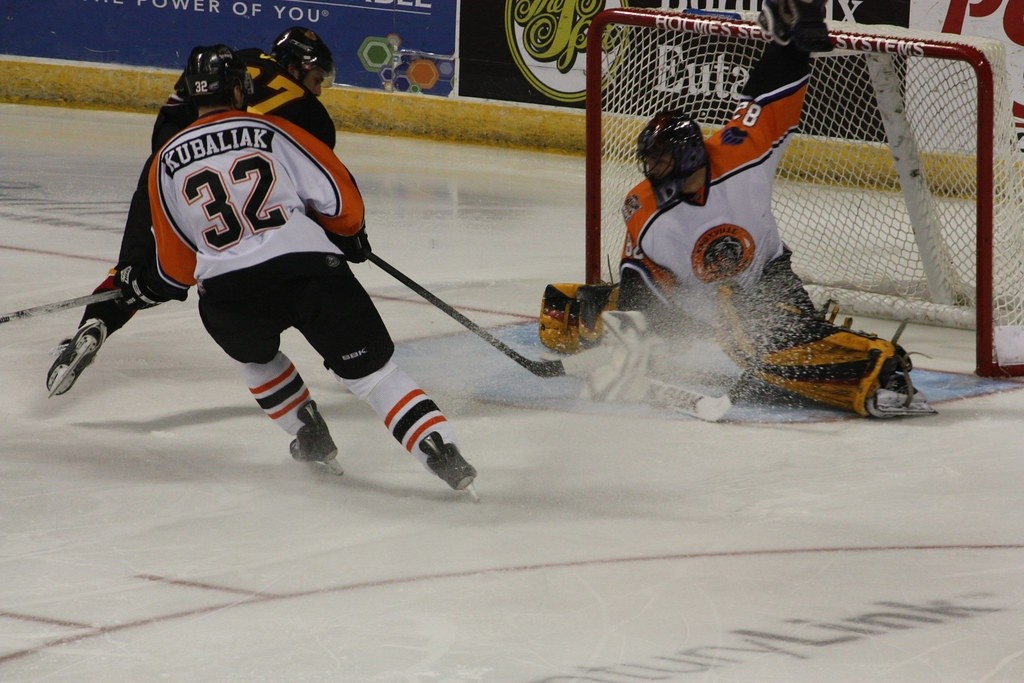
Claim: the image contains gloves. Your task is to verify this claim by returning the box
[336,230,372,264]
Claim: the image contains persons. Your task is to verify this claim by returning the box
[537,0,939,422]
[45,24,480,502]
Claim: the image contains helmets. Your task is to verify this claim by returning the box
[639,110,705,187]
[185,44,254,98]
[272,26,334,77]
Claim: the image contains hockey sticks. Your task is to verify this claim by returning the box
[366,253,616,378]
[0,287,123,325]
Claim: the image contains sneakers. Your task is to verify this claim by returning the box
[46,318,107,399]
[867,373,938,418]
[289,399,344,476]
[419,431,481,503]
[895,346,913,372]
